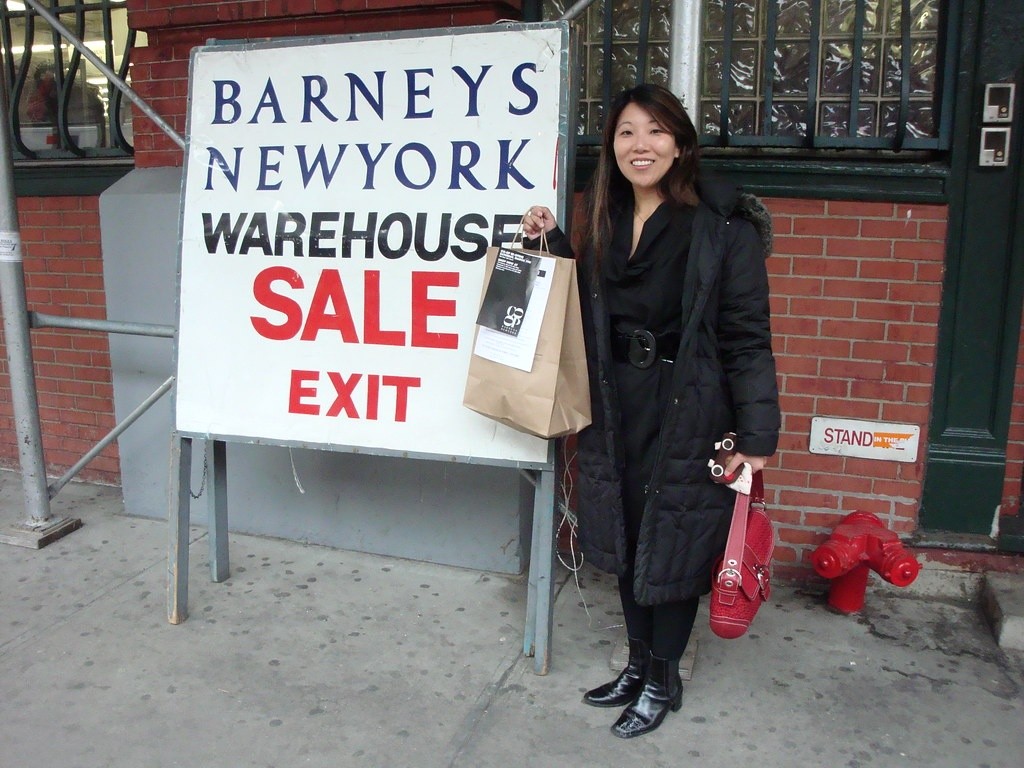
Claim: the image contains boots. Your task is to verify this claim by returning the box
[610,649,683,739]
[584,635,652,707]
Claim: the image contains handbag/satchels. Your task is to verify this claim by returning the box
[462,206,591,439]
[708,463,775,639]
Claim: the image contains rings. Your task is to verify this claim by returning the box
[528,211,531,216]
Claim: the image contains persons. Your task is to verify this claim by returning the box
[34,63,106,148]
[524,85,782,738]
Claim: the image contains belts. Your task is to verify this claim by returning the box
[610,322,680,367]
